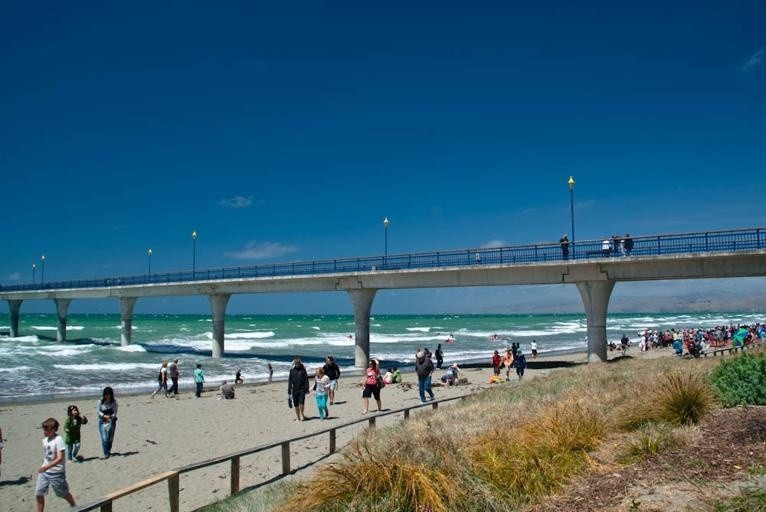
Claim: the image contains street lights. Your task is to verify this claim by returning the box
[383,218,390,269]
[567,174,576,259]
[147,247,152,284]
[190,230,197,280]
[40,256,45,285]
[30,262,37,283]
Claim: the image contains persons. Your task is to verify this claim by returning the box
[309,366,333,421]
[392,367,401,383]
[322,354,340,406]
[96,387,119,460]
[601,236,611,260]
[0,425,4,488]
[285,354,309,422]
[219,382,224,399]
[167,358,181,394]
[192,363,204,399]
[383,368,392,384]
[613,233,621,252]
[235,369,244,386]
[151,361,168,399]
[559,232,571,261]
[415,352,435,403]
[416,329,538,388]
[362,358,381,414]
[222,380,235,399]
[63,406,87,463]
[623,232,634,258]
[584,333,589,348]
[267,363,273,383]
[346,333,353,342]
[34,418,76,512]
[608,319,766,358]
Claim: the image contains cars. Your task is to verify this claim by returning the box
[104,276,122,286]
